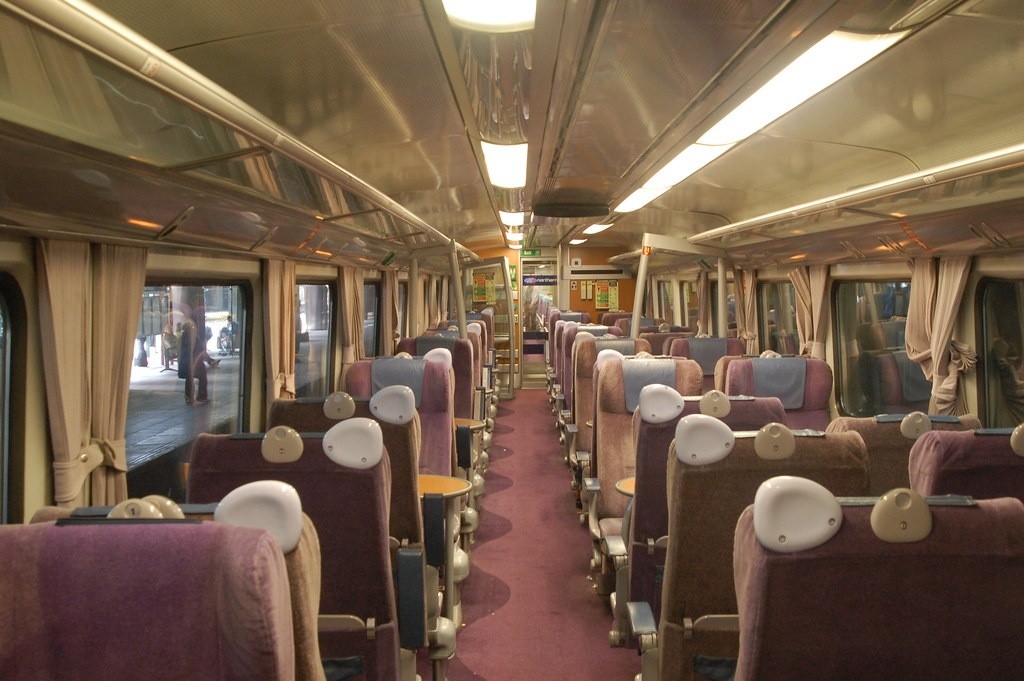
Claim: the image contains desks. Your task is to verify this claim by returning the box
[416,469,473,499]
[616,475,635,495]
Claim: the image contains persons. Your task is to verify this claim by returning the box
[174,311,240,367]
[523,286,539,330]
[177,320,211,405]
[295,299,303,363]
[162,324,179,356]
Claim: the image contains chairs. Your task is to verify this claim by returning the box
[824,412,982,498]
[0,519,296,681]
[340,305,502,581]
[545,307,836,573]
[189,417,419,680]
[30,480,330,680]
[730,476,1024,681]
[603,384,786,647]
[623,414,872,681]
[536,292,552,332]
[269,386,449,641]
[909,425,1024,500]
